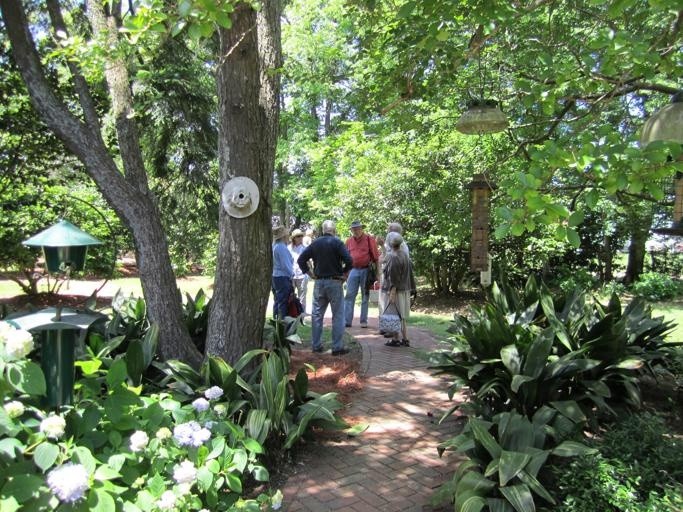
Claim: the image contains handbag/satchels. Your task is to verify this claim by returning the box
[365,262,381,290]
[288,298,304,317]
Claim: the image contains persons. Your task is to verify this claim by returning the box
[339,218,377,327]
[377,223,409,265]
[271,224,293,319]
[284,227,309,311]
[378,231,413,349]
[297,218,354,355]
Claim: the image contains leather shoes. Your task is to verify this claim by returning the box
[313,346,323,352]
[331,346,350,355]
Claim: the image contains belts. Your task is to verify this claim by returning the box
[351,266,369,269]
[315,275,340,279]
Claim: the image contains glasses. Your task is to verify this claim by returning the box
[297,235,304,237]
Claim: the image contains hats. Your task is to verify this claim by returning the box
[220,176,260,219]
[289,228,305,243]
[272,225,288,242]
[347,221,367,230]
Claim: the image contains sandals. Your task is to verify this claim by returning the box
[384,338,410,347]
